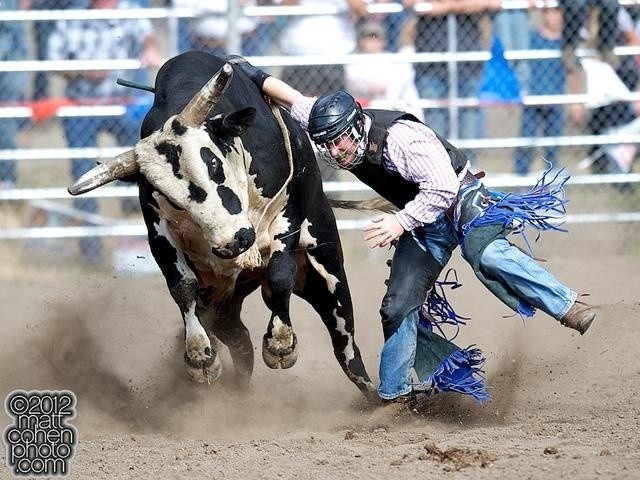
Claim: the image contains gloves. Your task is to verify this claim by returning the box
[219,53,272,91]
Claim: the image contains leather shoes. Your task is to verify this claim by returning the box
[559,300,597,336]
[370,393,417,428]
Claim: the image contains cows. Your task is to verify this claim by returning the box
[67,48,398,410]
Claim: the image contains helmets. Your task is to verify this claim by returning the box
[307,89,366,145]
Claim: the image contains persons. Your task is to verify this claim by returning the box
[226,52,594,432]
[0,0,640,256]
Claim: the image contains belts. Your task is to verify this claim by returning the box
[459,168,487,189]
